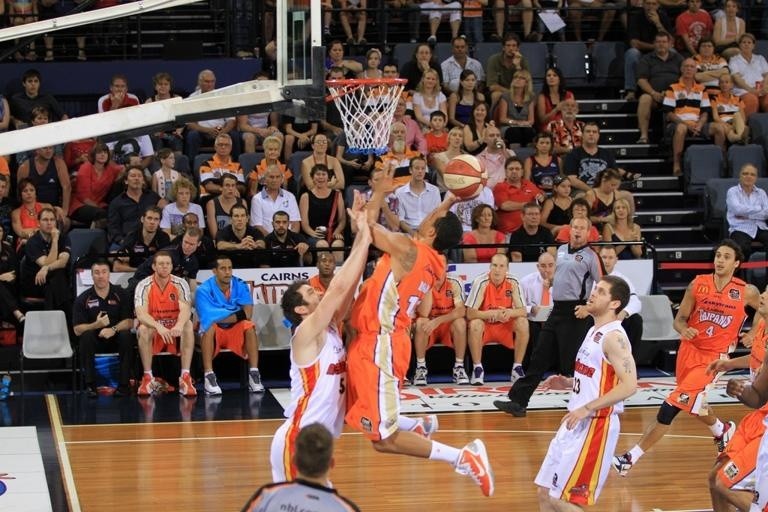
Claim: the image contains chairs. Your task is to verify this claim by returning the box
[639,295,682,341]
[193,153,214,183]
[703,177,738,228]
[727,143,765,178]
[432,43,453,65]
[148,154,190,176]
[518,42,548,87]
[238,153,265,178]
[345,185,368,209]
[589,41,629,87]
[252,304,292,352]
[683,144,726,196]
[753,178,768,196]
[20,310,76,396]
[513,148,537,163]
[473,43,503,74]
[393,43,429,73]
[551,41,589,87]
[67,228,107,277]
[287,151,312,181]
[748,113,768,143]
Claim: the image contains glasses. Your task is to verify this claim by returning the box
[312,140,327,144]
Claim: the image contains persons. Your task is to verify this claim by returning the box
[708,355,767,512]
[1,1,768,402]
[270,206,375,490]
[610,243,762,479]
[710,284,768,387]
[238,424,358,512]
[532,277,636,511]
[493,217,610,416]
[342,161,496,501]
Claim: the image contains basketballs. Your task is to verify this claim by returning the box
[443,153,488,200]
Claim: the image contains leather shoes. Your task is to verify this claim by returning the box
[87,385,98,398]
[112,385,130,398]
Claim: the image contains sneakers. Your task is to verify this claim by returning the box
[137,373,155,396]
[248,371,264,394]
[713,421,737,458]
[178,372,197,397]
[203,371,222,396]
[493,400,527,418]
[413,363,525,386]
[611,451,633,478]
[453,438,495,498]
[408,414,439,440]
